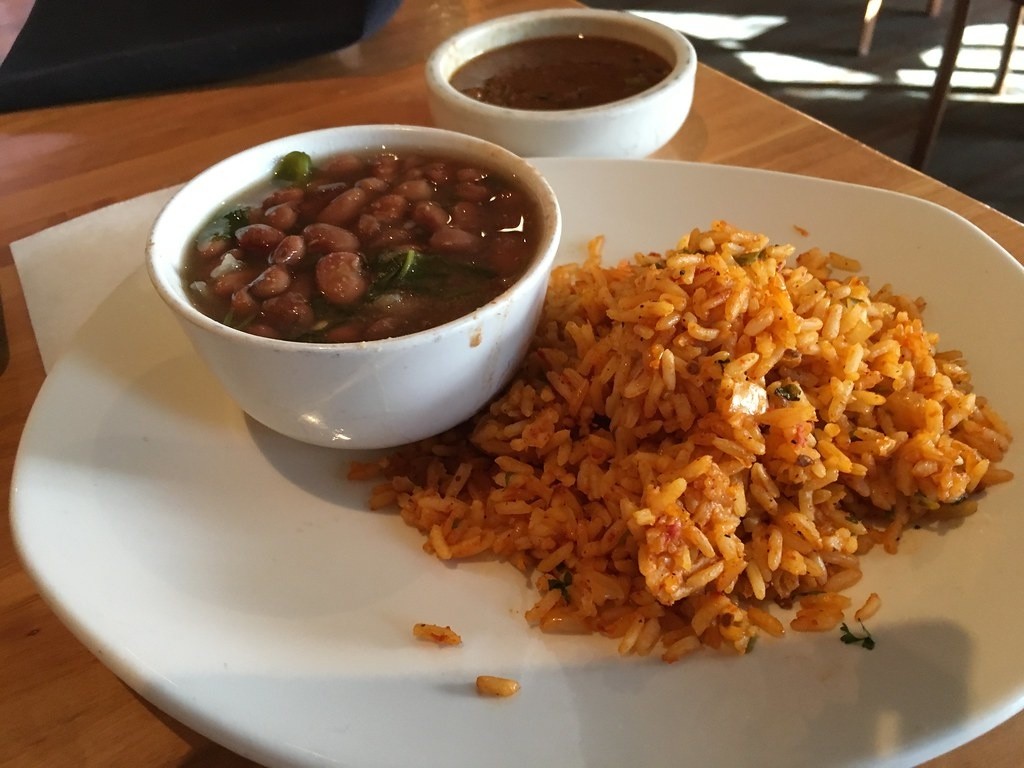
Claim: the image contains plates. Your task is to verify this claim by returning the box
[10,150,1023,768]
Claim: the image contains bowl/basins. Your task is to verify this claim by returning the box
[144,121,565,453]
[425,6,698,163]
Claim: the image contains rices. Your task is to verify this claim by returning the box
[327,209,1016,713]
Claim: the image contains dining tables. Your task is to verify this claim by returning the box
[3,0,1023,768]
